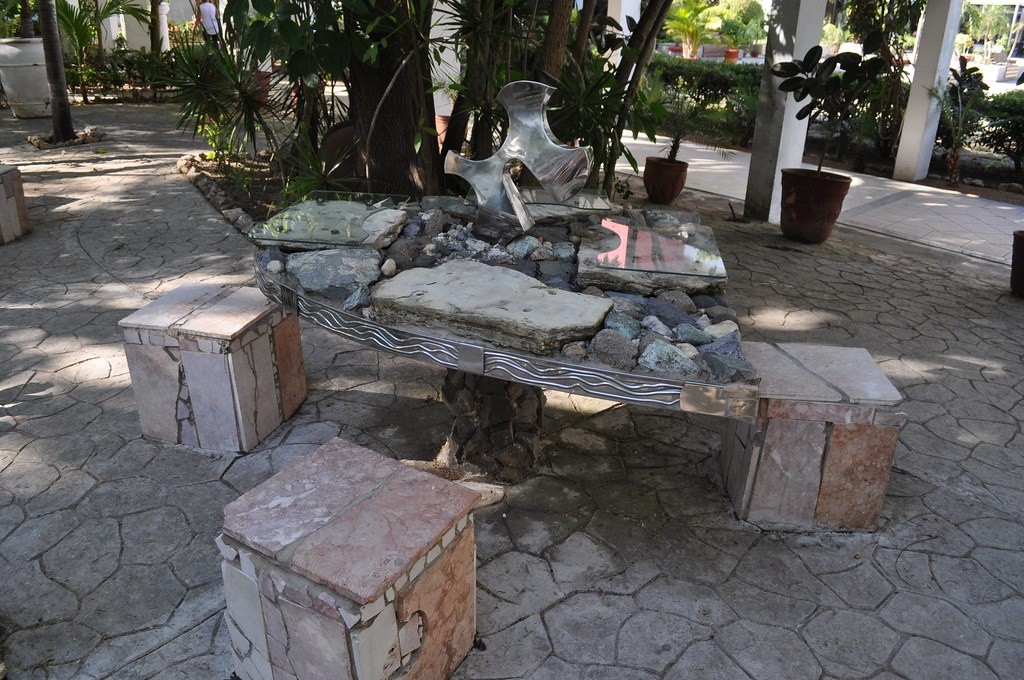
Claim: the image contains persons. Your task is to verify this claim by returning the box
[195,0,219,45]
[429,0,473,147]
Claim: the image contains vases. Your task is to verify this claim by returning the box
[436,115,469,146]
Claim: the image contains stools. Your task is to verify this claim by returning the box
[117,282,308,452]
[212,434,483,680]
[0,164,33,246]
[721,341,907,534]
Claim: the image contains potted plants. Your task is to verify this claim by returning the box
[767,28,886,244]
[633,50,760,207]
[0,0,51,119]
